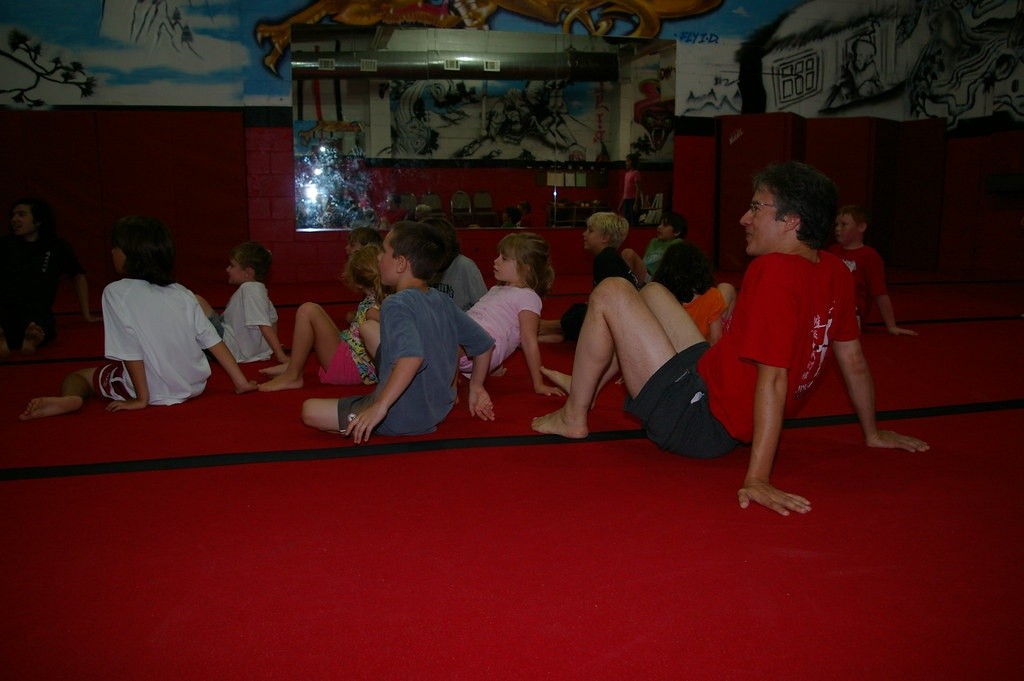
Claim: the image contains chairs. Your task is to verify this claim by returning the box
[388,192,496,229]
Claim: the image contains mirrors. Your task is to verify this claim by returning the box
[290,22,677,233]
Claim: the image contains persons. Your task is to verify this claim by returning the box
[19,215,259,421]
[277,213,737,446]
[531,157,930,516]
[0,197,101,357]
[319,194,529,227]
[617,153,642,226]
[196,241,290,363]
[826,206,920,336]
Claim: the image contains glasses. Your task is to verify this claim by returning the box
[750,201,786,215]
